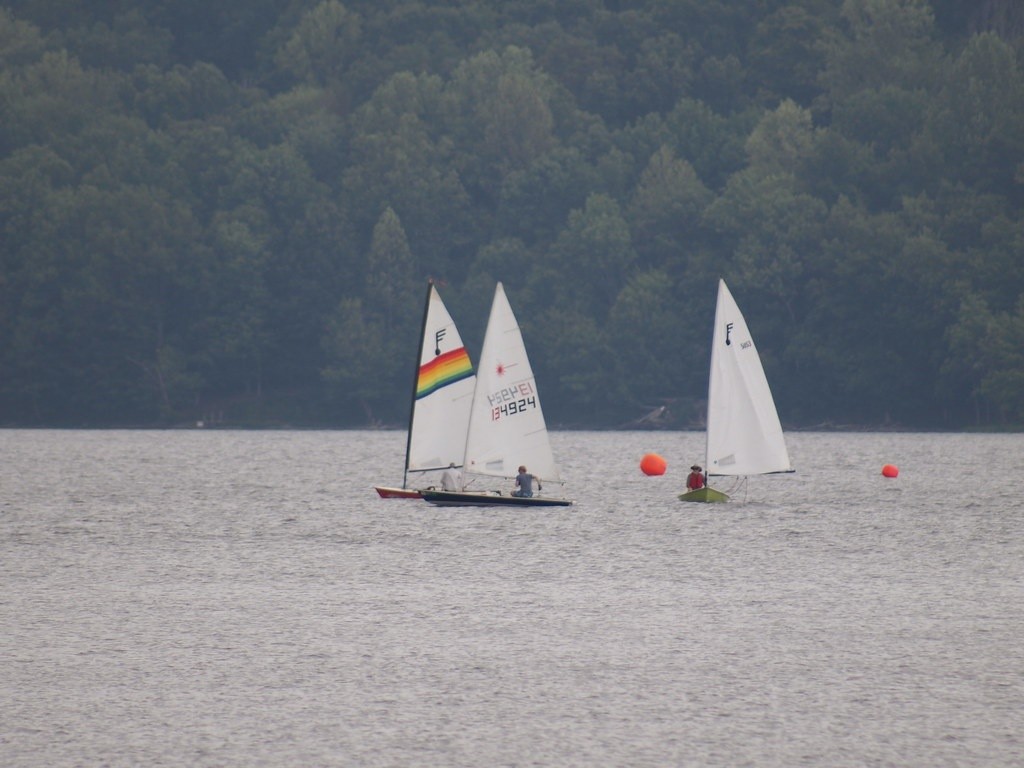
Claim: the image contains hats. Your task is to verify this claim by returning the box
[690,463,702,472]
[448,463,458,468]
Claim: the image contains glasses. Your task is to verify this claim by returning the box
[518,470,520,472]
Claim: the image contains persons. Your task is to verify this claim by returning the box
[686,463,706,492]
[440,463,462,492]
[510,465,542,498]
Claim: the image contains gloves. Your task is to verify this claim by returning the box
[518,481,520,485]
[538,484,542,491]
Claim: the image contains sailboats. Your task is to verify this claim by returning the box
[417,281,573,508]
[374,278,503,499]
[678,278,796,502]
